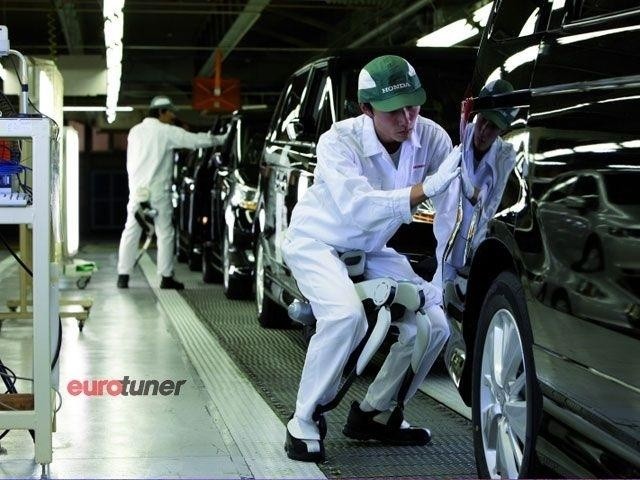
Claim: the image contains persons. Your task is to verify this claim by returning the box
[279,54,463,463]
[116,96,230,291]
[441,78,517,313]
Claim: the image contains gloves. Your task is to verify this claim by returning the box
[216,129,229,145]
[424,141,464,198]
[461,162,475,200]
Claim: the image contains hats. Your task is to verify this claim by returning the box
[357,54,427,114]
[149,96,175,111]
[479,81,520,130]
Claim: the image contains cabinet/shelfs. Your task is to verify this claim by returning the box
[0,113,61,479]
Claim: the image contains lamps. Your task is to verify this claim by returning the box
[63,0,134,124]
[416,0,495,47]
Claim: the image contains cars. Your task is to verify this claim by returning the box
[173,0,640,480]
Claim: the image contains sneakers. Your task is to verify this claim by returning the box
[343,398,433,446]
[160,277,184,290]
[284,414,326,463]
[117,274,131,287]
